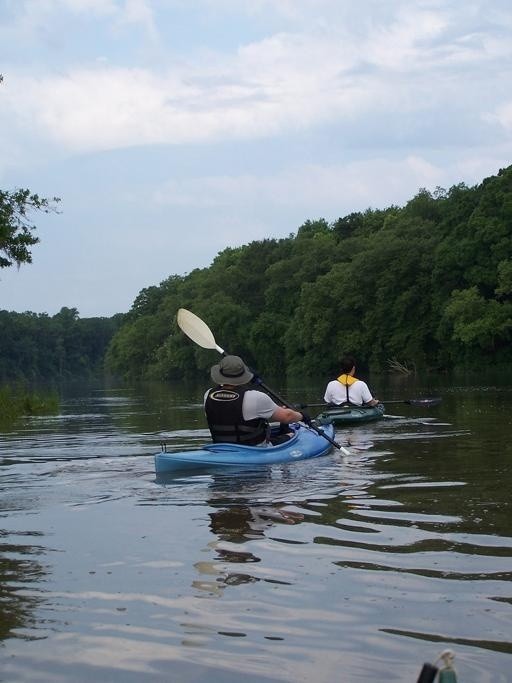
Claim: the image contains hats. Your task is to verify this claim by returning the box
[210,355,254,386]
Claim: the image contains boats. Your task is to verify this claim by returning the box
[315,404,385,426]
[154,422,335,479]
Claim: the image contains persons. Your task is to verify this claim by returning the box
[322,358,380,407]
[201,353,312,448]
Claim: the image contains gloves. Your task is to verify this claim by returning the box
[300,411,311,423]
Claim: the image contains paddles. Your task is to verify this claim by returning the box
[178,308,351,456]
[287,398,442,405]
